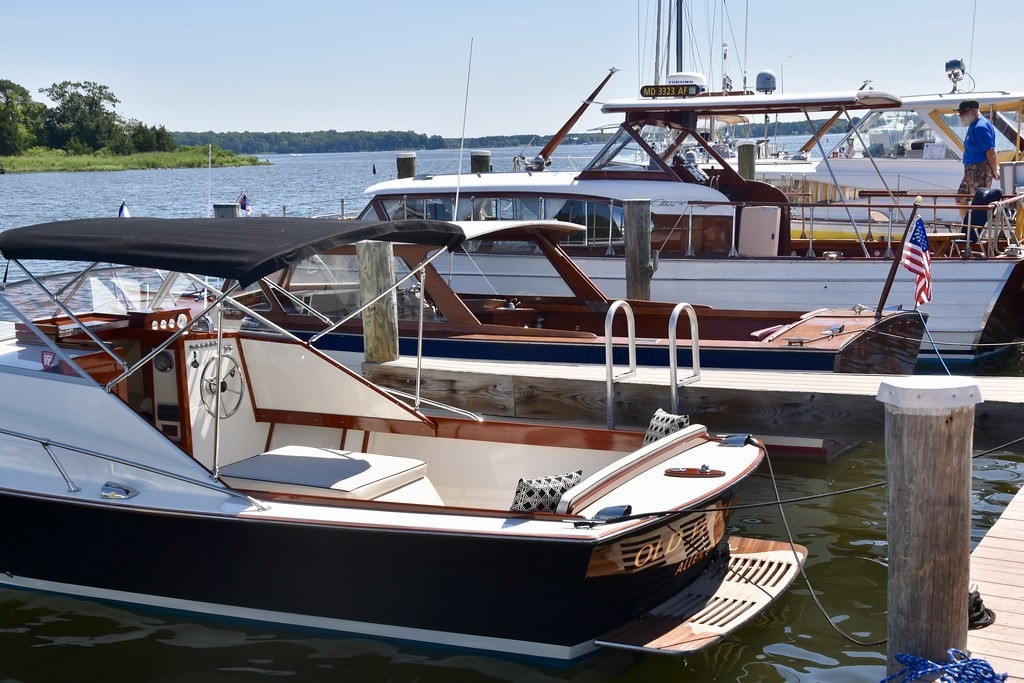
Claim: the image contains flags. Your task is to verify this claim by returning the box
[239,196,253,216]
[900,214,933,308]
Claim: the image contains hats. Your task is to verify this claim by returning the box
[954,101,980,113]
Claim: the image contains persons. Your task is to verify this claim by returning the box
[953,99,1000,233]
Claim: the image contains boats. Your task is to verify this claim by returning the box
[261,1,1024,375]
[0,142,810,673]
